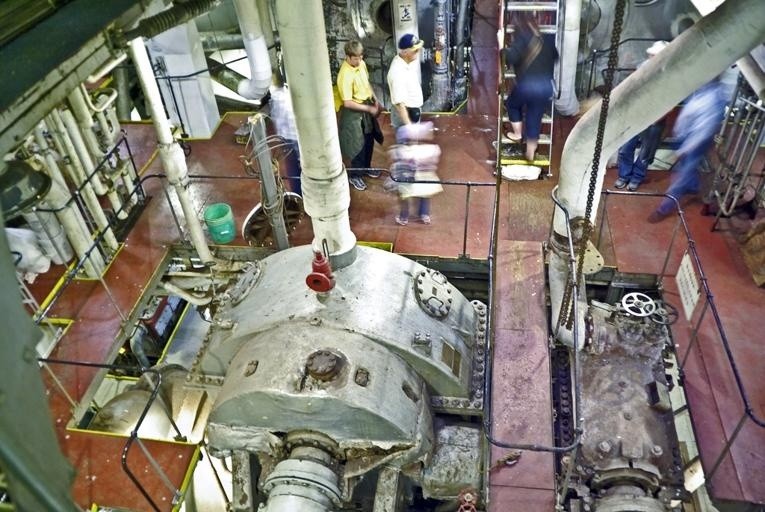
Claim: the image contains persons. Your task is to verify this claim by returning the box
[269,56,302,196]
[614,40,672,190]
[384,121,442,226]
[501,9,559,162]
[336,37,383,191]
[646,77,724,223]
[384,34,426,129]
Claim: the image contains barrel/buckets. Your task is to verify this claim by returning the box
[203,203,235,244]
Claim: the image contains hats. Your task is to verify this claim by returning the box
[399,34,425,52]
[647,39,670,54]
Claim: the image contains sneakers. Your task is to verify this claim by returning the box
[395,215,409,225]
[364,167,382,178]
[523,152,535,161]
[648,211,667,223]
[615,178,626,189]
[625,182,639,190]
[349,176,367,191]
[420,214,431,224]
[504,130,523,144]
